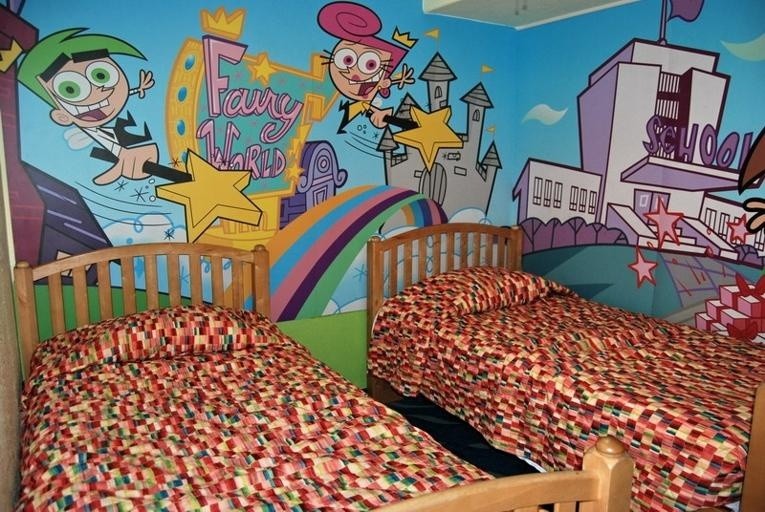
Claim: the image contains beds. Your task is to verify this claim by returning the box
[367,224,764,512]
[12,242,634,511]
